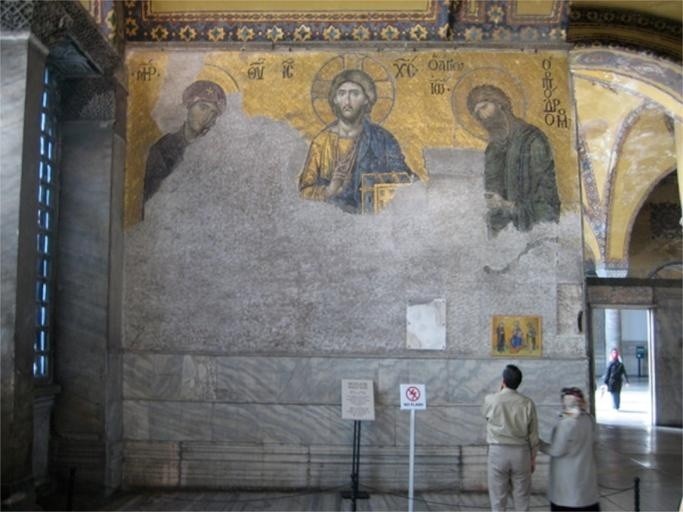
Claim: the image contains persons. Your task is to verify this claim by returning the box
[482,364,541,512]
[603,351,630,411]
[298,69,420,218]
[467,83,561,241]
[535,387,601,511]
[139,80,227,221]
